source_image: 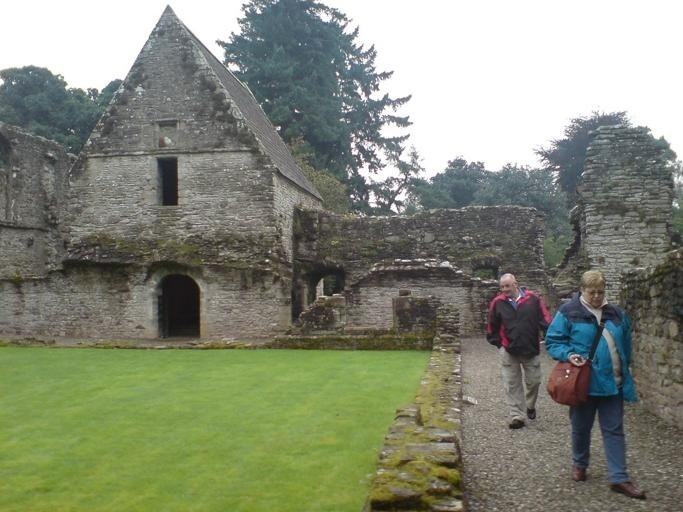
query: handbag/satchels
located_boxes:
[547,361,591,407]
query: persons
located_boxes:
[545,270,646,499]
[486,273,552,428]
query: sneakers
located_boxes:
[509,419,524,429]
[527,407,536,420]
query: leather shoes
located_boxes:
[571,464,586,482]
[608,481,645,499]
